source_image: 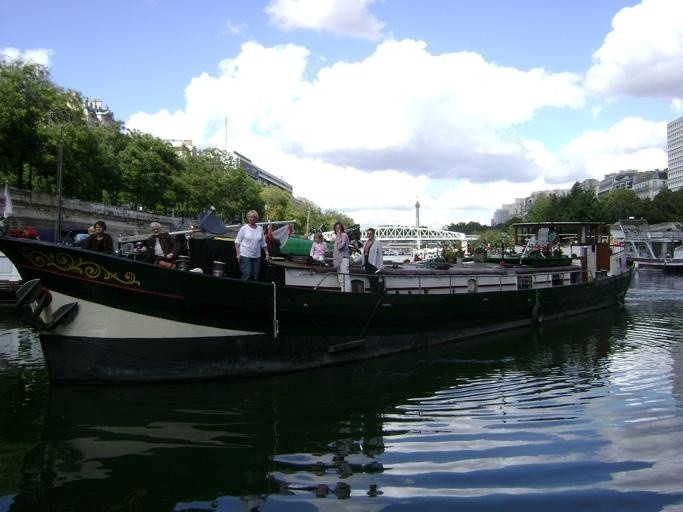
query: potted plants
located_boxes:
[484,230,513,268]
[446,252,455,263]
[456,250,464,265]
[473,247,487,262]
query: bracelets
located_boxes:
[264,251,268,255]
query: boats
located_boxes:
[0,223,637,384]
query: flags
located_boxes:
[2,185,13,220]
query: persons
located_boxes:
[233,209,271,280]
[74,224,95,245]
[360,228,384,293]
[330,222,352,293]
[304,405,388,501]
[306,230,328,268]
[136,221,175,267]
[70,221,114,256]
